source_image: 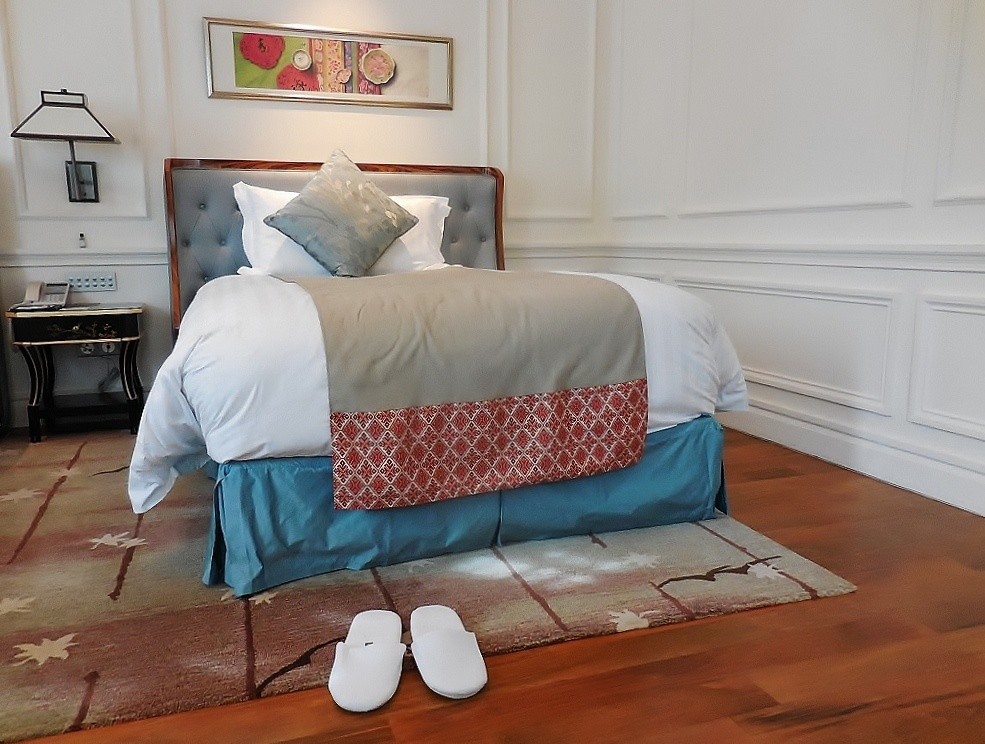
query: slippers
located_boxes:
[328,610,407,711]
[409,605,488,699]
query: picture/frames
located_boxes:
[202,16,455,112]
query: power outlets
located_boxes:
[75,343,100,359]
[99,342,120,356]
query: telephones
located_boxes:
[23,280,70,307]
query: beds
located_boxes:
[124,148,749,599]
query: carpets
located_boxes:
[0,417,862,744]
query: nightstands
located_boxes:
[4,302,147,444]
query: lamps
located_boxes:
[8,88,115,204]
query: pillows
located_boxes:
[231,180,452,285]
[262,148,420,278]
